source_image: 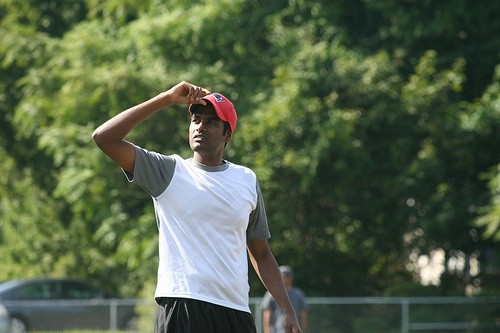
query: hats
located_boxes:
[279,265,294,274]
[189,93,238,134]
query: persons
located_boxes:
[91,81,302,333]
[259,265,308,333]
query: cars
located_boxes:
[0,277,141,333]
